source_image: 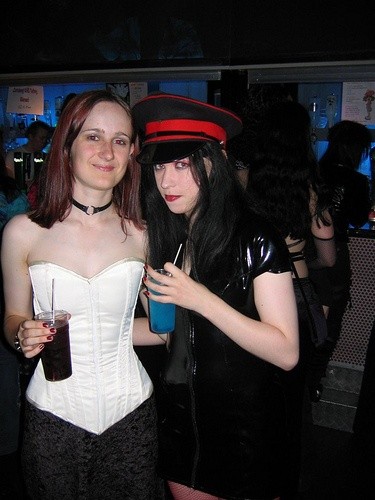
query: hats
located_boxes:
[130,92,245,167]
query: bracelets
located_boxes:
[14,323,24,355]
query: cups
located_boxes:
[146,269,176,334]
[33,310,72,381]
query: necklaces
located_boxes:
[68,191,114,215]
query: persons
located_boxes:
[0,154,31,231]
[225,82,294,190]
[235,96,335,500]
[0,90,171,500]
[308,119,372,402]
[4,122,49,181]
[132,96,300,500]
